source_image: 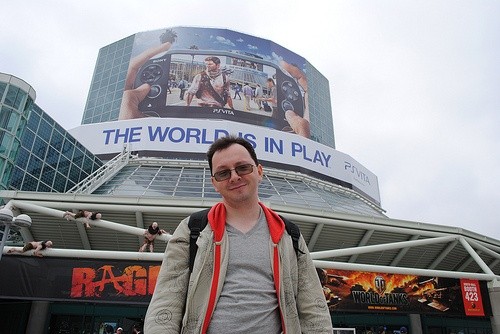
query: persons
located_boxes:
[367,326,407,334]
[167,77,188,100]
[143,137,333,334]
[118,41,310,139]
[186,57,235,109]
[100,324,143,334]
[243,82,254,111]
[234,83,242,100]
[63,210,102,228]
[7,240,52,256]
[139,222,166,252]
[255,78,277,116]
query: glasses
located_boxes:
[212,164,255,183]
